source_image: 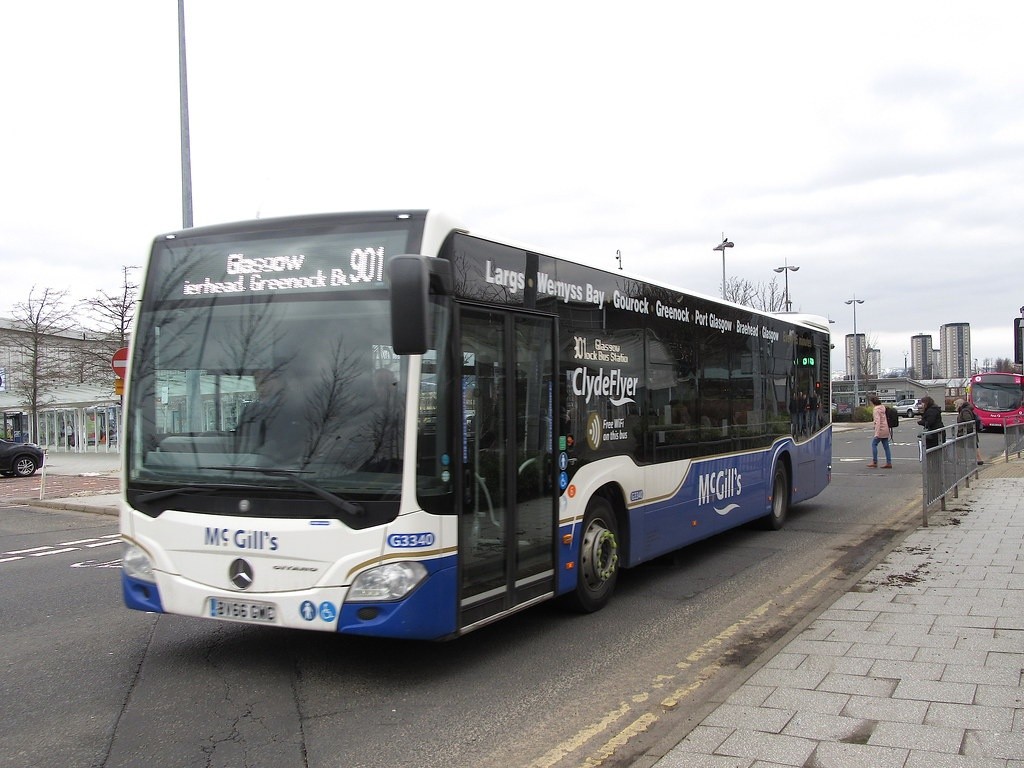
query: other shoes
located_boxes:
[977,460,984,465]
[866,459,877,467]
[881,462,892,469]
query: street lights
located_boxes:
[844,294,864,408]
[712,231,734,300]
[615,249,622,270]
[773,257,800,312]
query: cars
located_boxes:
[0,438,44,478]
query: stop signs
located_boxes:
[112,347,128,379]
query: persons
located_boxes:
[670,400,689,424]
[790,390,818,439]
[916,397,946,448]
[236,367,291,438]
[866,396,892,468]
[68,421,73,445]
[954,399,983,466]
[372,370,397,393]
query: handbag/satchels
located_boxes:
[885,406,898,428]
[917,423,931,440]
[974,413,981,431]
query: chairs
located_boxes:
[622,394,757,438]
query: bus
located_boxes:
[832,391,879,414]
[0,409,105,450]
[965,373,1024,434]
[116,206,833,636]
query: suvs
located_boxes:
[892,398,926,418]
